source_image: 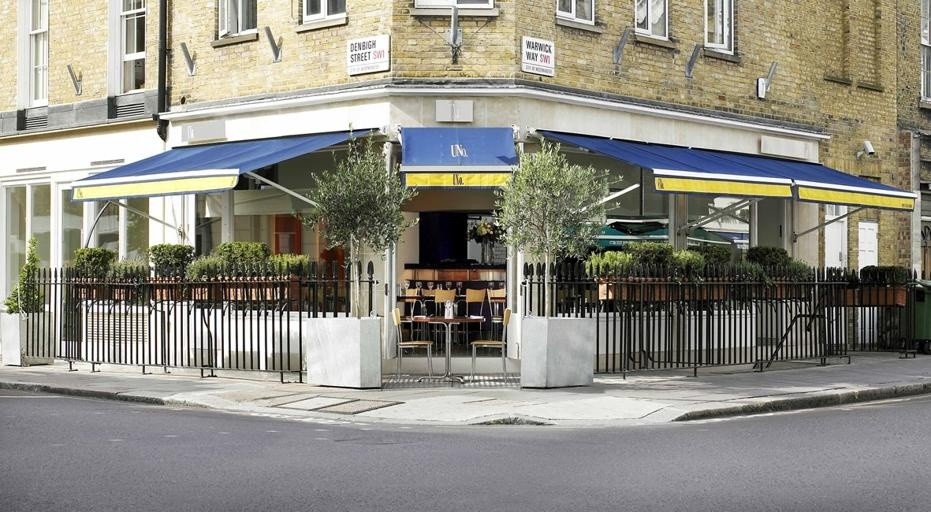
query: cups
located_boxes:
[498,282,505,289]
[416,282,422,288]
[437,283,443,290]
[403,281,410,289]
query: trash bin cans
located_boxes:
[900,278,931,355]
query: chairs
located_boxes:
[390,287,511,385]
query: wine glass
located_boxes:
[446,281,453,290]
[488,282,494,290]
[456,282,462,297]
[427,282,434,291]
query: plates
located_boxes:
[468,315,485,319]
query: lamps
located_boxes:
[263,25,281,64]
[66,63,82,96]
[756,61,777,100]
[612,27,630,66]
[449,5,461,64]
[684,44,702,79]
[178,42,196,77]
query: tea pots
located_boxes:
[444,300,454,320]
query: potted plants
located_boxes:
[490,138,622,390]
[305,125,422,392]
[1,239,53,367]
[73,241,316,303]
